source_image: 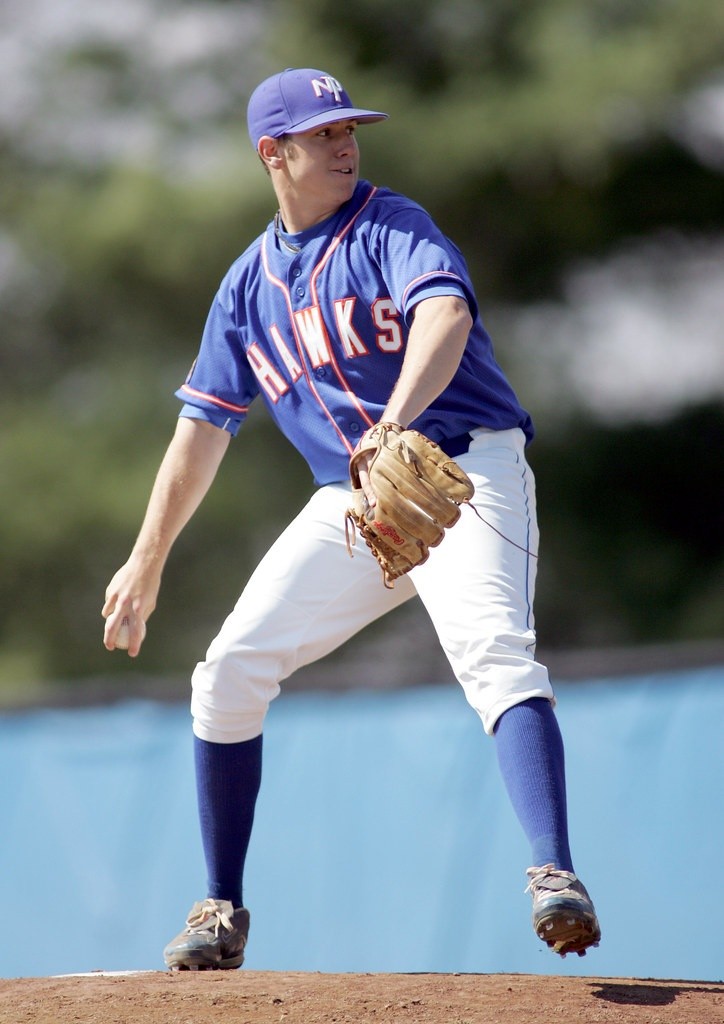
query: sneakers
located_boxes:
[525,864,601,959]
[163,900,250,972]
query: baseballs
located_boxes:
[106,613,146,650]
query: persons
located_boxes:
[101,68,600,972]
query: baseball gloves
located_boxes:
[341,424,476,591]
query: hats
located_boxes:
[247,68,388,150]
